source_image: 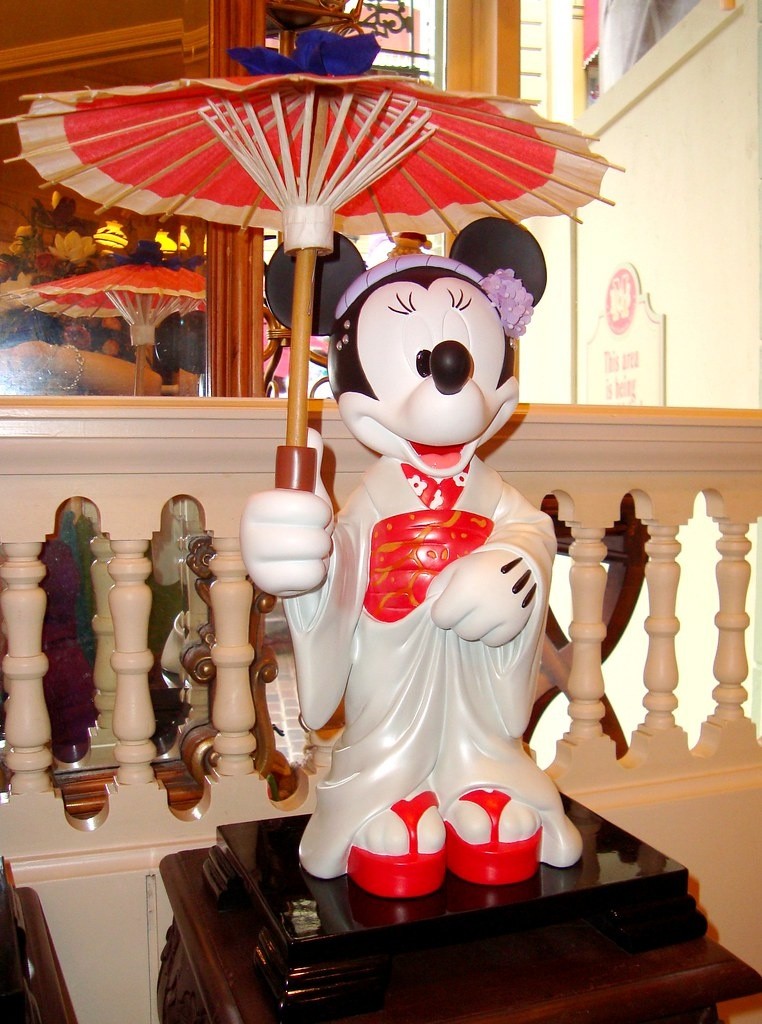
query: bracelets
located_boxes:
[29,344,85,391]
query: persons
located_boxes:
[1,306,161,395]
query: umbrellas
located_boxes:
[2,29,628,493]
[1,240,207,395]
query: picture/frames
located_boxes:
[572,0,742,144]
